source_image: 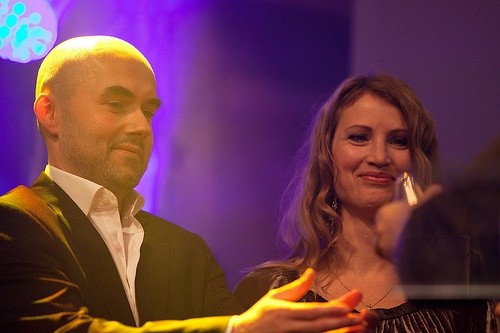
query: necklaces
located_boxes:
[328,256,408,308]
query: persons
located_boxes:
[375,137,499,315]
[233,74,499,333]
[0,33,377,332]
